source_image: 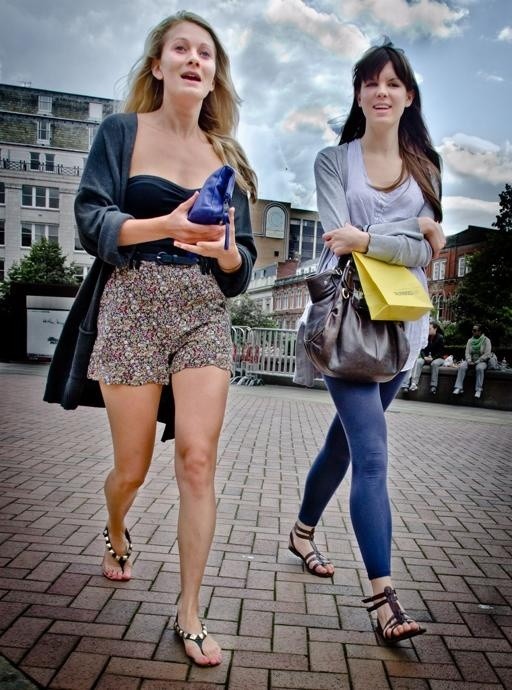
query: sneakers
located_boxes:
[401,381,439,396]
[452,387,481,399]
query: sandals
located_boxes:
[174,612,222,668]
[287,520,336,578]
[100,523,134,583]
[360,586,430,643]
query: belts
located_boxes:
[129,249,200,266]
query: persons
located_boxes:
[452,324,492,399]
[409,321,448,394]
[288,36,448,644]
[401,378,411,393]
[41,9,257,666]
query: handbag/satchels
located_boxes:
[186,164,237,228]
[350,249,434,322]
[487,352,501,370]
[302,244,410,385]
